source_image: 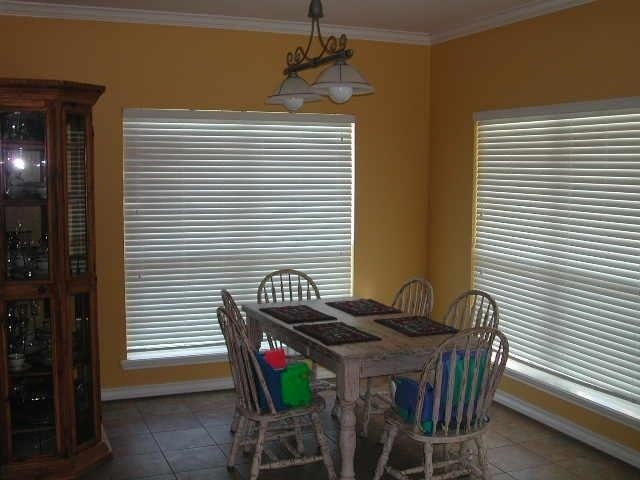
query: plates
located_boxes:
[5,363,32,375]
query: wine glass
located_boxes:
[5,297,53,363]
[3,218,51,283]
[2,109,51,199]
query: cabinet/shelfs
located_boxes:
[0,75,112,480]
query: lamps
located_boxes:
[264,0,375,112]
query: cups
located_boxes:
[263,349,288,372]
[8,353,26,369]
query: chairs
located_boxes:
[373,327,509,480]
[256,269,321,374]
[361,290,499,445]
[216,308,338,480]
[221,288,256,455]
[391,278,434,317]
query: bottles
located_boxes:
[11,384,52,427]
[12,430,56,459]
[72,311,90,391]
